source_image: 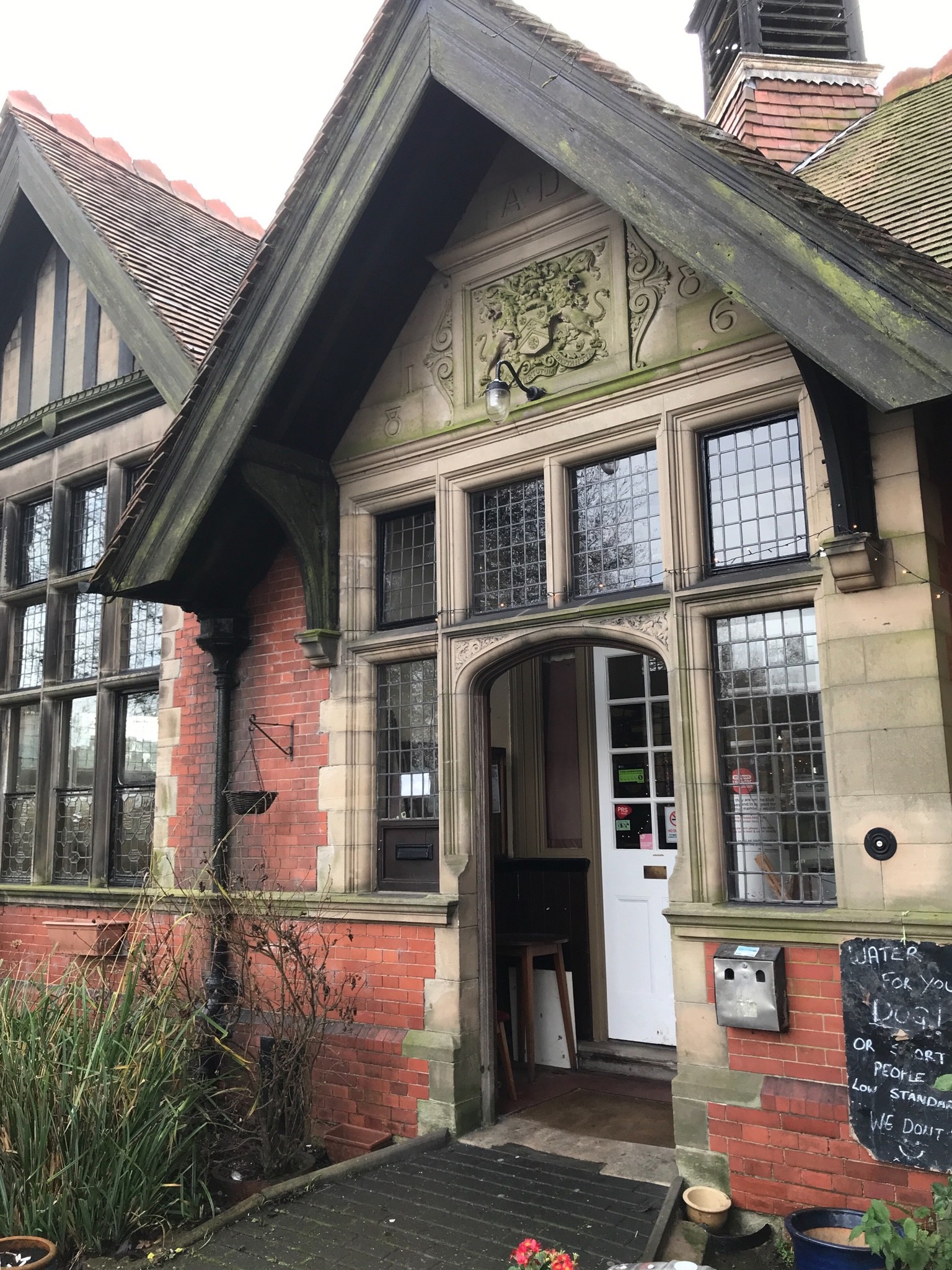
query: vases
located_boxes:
[786,1208,903,1269]
[683,1188,730,1231]
[326,1124,393,1163]
[42,918,128,956]
[0,1236,56,1270]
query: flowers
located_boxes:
[509,1235,578,1268]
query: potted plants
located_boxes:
[210,874,317,1198]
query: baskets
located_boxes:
[223,790,278,814]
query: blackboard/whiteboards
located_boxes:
[841,937,952,1175]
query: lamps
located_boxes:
[487,361,541,426]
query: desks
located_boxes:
[496,931,578,1084]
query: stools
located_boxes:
[496,1011,517,1100]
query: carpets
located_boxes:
[504,1086,675,1150]
